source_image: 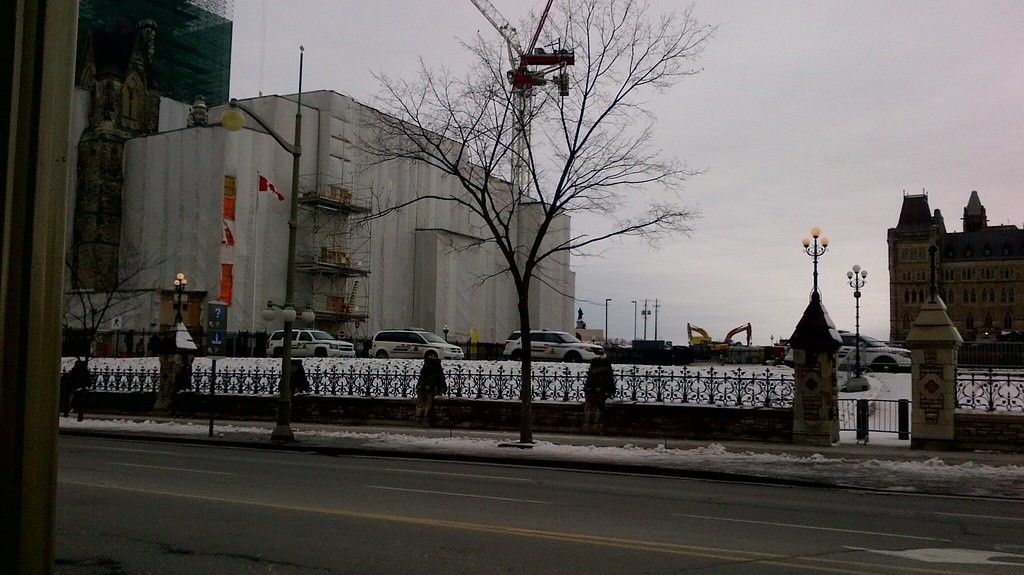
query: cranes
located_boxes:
[470,1,575,197]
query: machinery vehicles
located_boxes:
[686,322,752,360]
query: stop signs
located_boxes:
[338,330,346,339]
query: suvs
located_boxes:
[503,330,607,363]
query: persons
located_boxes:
[720,348,725,366]
[576,332,581,342]
[124,329,176,358]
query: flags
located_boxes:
[256,173,285,201]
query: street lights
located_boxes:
[847,264,870,392]
[631,301,637,341]
[172,273,188,326]
[801,227,829,302]
[605,298,611,350]
[221,45,315,443]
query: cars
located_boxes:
[957,341,1024,365]
[783,329,912,373]
[368,328,465,361]
[265,327,356,357]
